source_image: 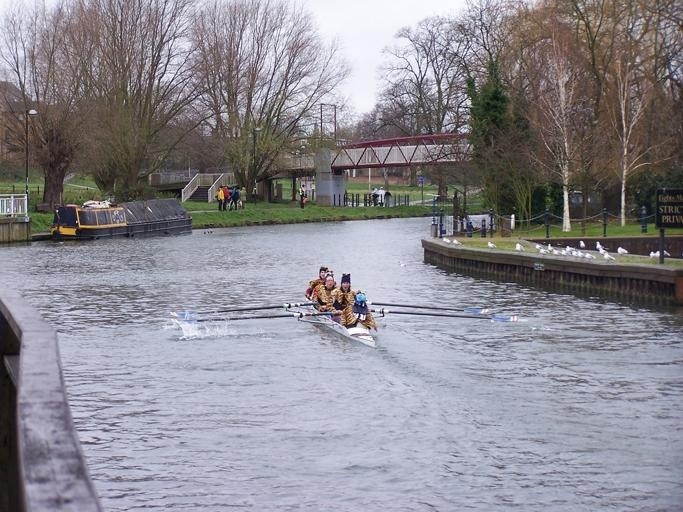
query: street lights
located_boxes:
[250,127,261,205]
[23,108,38,213]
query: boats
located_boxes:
[51,197,193,240]
[303,296,379,351]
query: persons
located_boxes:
[306,267,375,329]
[299,184,306,208]
[251,185,258,205]
[217,184,248,211]
[370,186,392,207]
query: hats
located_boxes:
[341,274,350,282]
[356,293,366,302]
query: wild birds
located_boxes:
[595,241,609,250]
[452,239,463,247]
[535,240,596,260]
[515,243,526,253]
[617,246,629,256]
[603,251,615,263]
[442,237,452,246]
[649,250,671,260]
[487,242,498,250]
[599,247,608,255]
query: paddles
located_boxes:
[366,301,490,314]
[178,302,318,318]
[369,308,518,323]
[184,311,340,324]
